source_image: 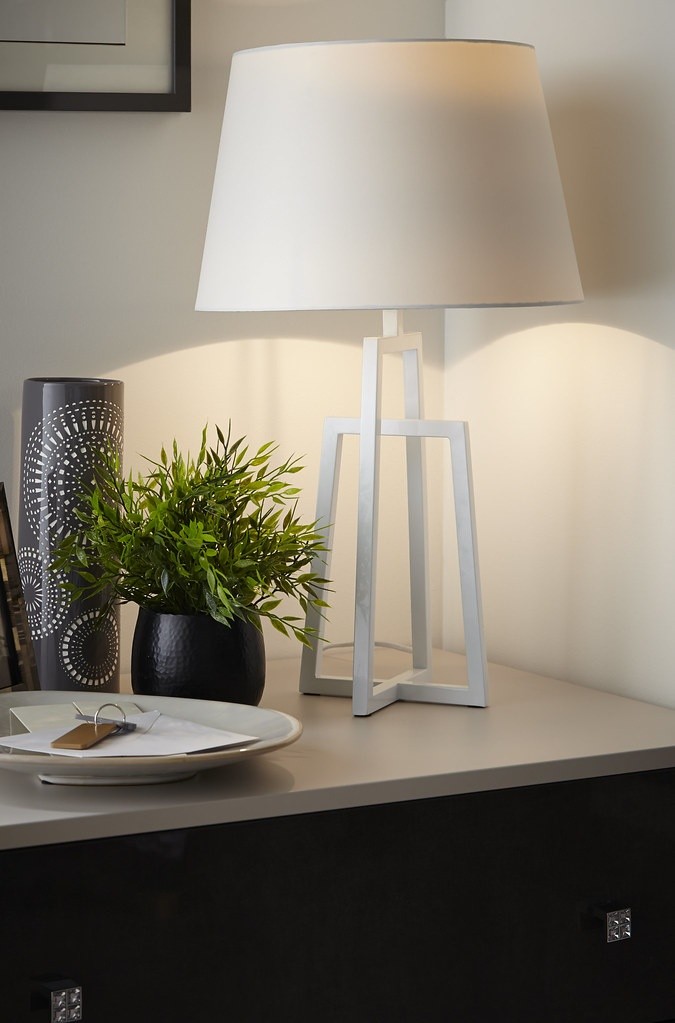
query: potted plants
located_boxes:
[47,420,337,708]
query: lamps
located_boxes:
[192,39,582,718]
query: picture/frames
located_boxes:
[0,0,193,113]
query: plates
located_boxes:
[1,691,304,786]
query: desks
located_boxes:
[0,641,675,1023]
